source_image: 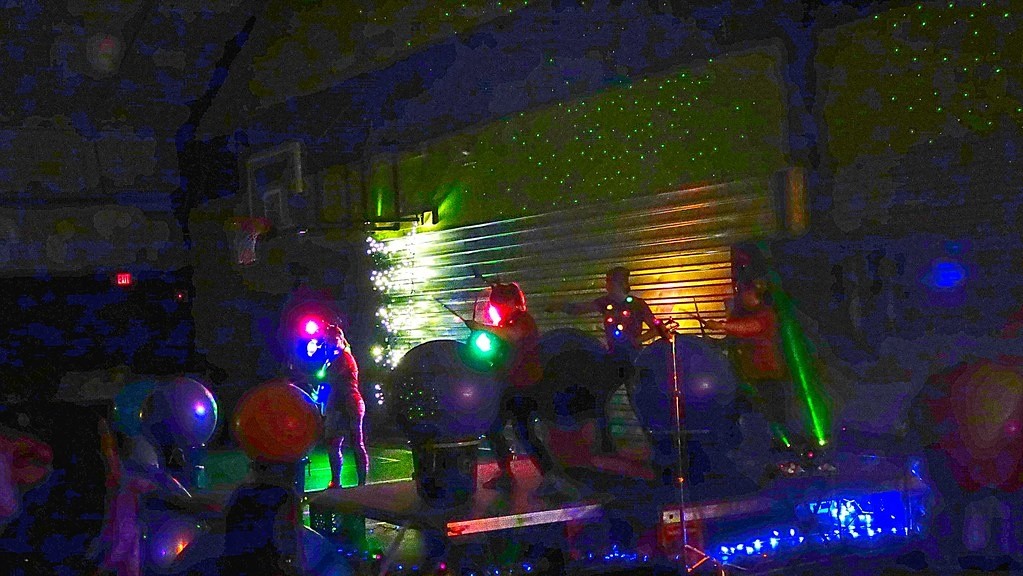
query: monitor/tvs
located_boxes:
[248,139,308,242]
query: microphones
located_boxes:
[636,321,672,347]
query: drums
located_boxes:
[408,441,480,509]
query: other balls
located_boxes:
[147,372,218,447]
[386,340,506,444]
[232,378,324,462]
[632,331,733,434]
[532,330,613,424]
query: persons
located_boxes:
[545,268,672,452]
[465,283,567,496]
[18,403,197,576]
[217,458,306,576]
[288,326,368,490]
[706,276,836,473]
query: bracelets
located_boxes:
[719,320,726,330]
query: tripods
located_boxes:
[662,333,756,576]
